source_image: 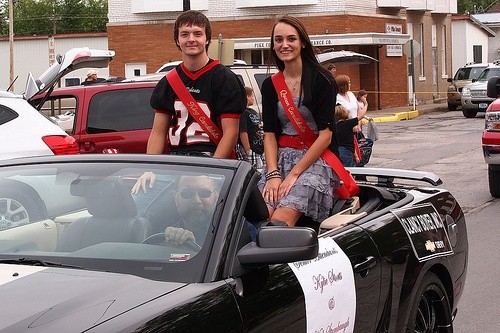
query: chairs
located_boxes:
[57,178,269,252]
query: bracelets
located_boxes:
[265,170,282,180]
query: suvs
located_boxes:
[447,62,496,111]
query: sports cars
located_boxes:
[0,153,469,333]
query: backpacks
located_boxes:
[356,131,373,167]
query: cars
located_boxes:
[120,48,378,134]
[27,81,175,154]
[0,47,115,161]
[461,60,500,118]
[481,77,500,197]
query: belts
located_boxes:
[278,135,309,150]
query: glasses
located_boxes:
[181,189,215,199]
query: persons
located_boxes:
[328,65,336,75]
[356,91,371,133]
[333,77,365,166]
[130,10,246,218]
[333,99,370,168]
[161,175,259,247]
[255,15,338,227]
[81,70,100,85]
[237,87,266,169]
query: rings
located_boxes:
[265,190,270,192]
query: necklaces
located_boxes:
[286,74,301,90]
